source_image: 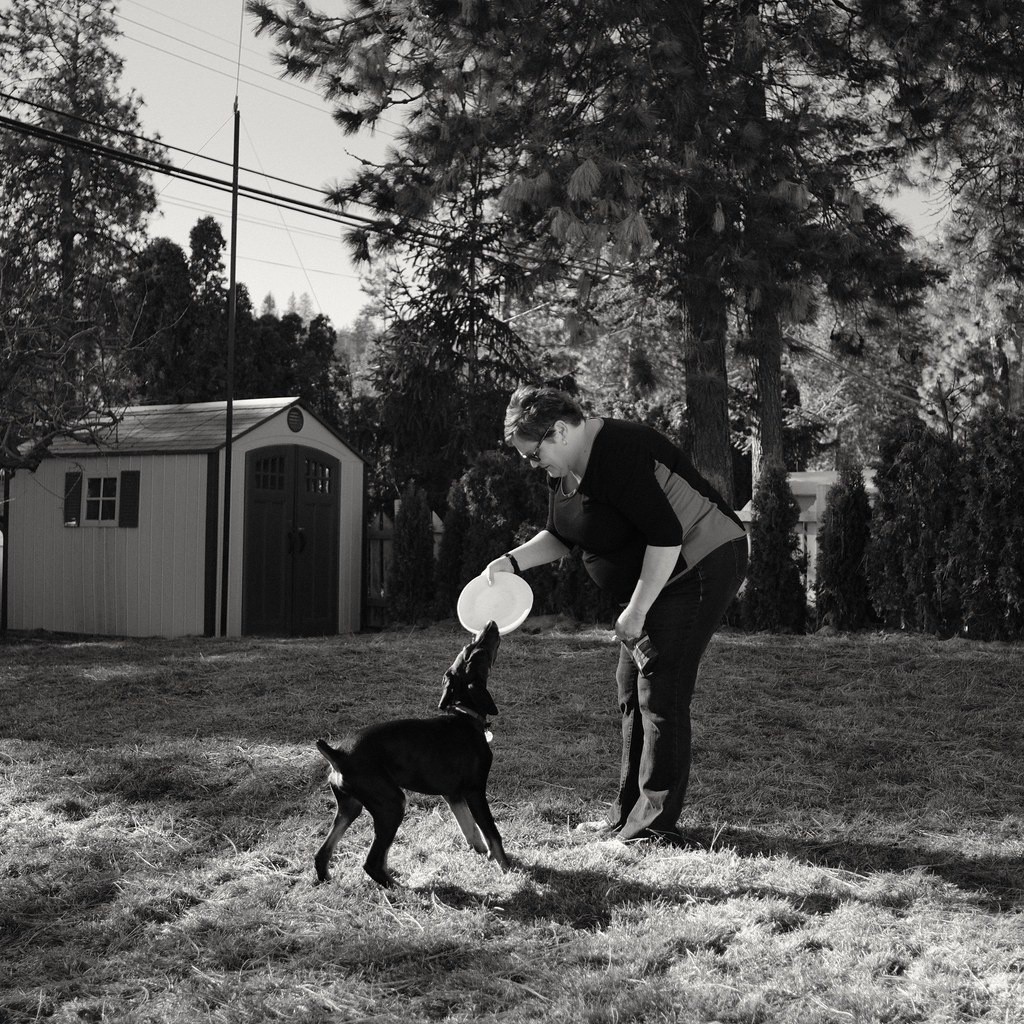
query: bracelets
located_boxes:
[503,553,522,574]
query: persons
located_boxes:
[479,384,749,853]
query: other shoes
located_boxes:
[576,820,611,836]
[596,840,630,855]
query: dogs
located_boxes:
[313,619,515,893]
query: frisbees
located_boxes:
[456,572,533,636]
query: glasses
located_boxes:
[522,423,555,464]
